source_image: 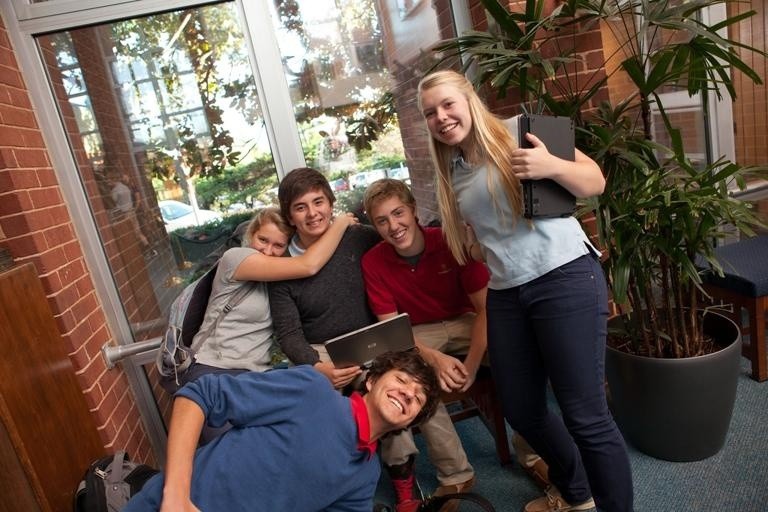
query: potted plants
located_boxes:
[420,0,768,463]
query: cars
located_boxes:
[324,167,413,193]
[159,199,222,235]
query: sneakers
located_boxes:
[433,475,474,512]
[390,456,420,512]
[523,459,597,512]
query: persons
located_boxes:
[364,178,555,511]
[179,209,354,443]
[116,350,441,512]
[416,70,633,510]
[99,169,160,259]
[270,169,424,512]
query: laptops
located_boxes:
[517,114,576,220]
[324,312,416,369]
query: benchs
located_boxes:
[687,234,768,382]
[412,355,514,473]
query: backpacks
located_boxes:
[156,259,220,392]
[74,451,160,512]
